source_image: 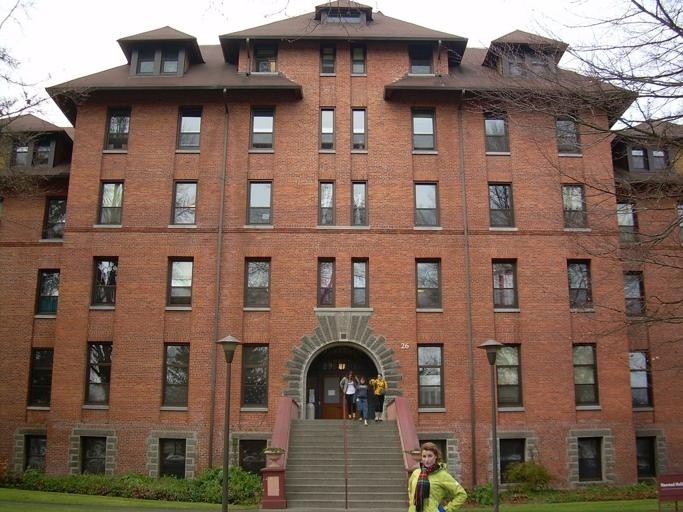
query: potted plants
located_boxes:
[400,448,422,468]
[263,447,287,467]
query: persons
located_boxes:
[405,442,468,512]
[368,373,387,421]
[354,375,372,425]
[339,370,360,418]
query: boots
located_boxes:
[374,411,383,421]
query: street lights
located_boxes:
[475,339,507,512]
[215,333,245,512]
